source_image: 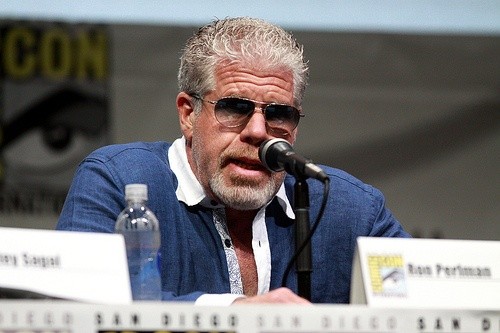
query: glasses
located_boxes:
[187,93,306,135]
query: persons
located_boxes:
[56,17,413,306]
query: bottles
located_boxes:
[114,184,162,301]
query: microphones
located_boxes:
[258,138,330,184]
